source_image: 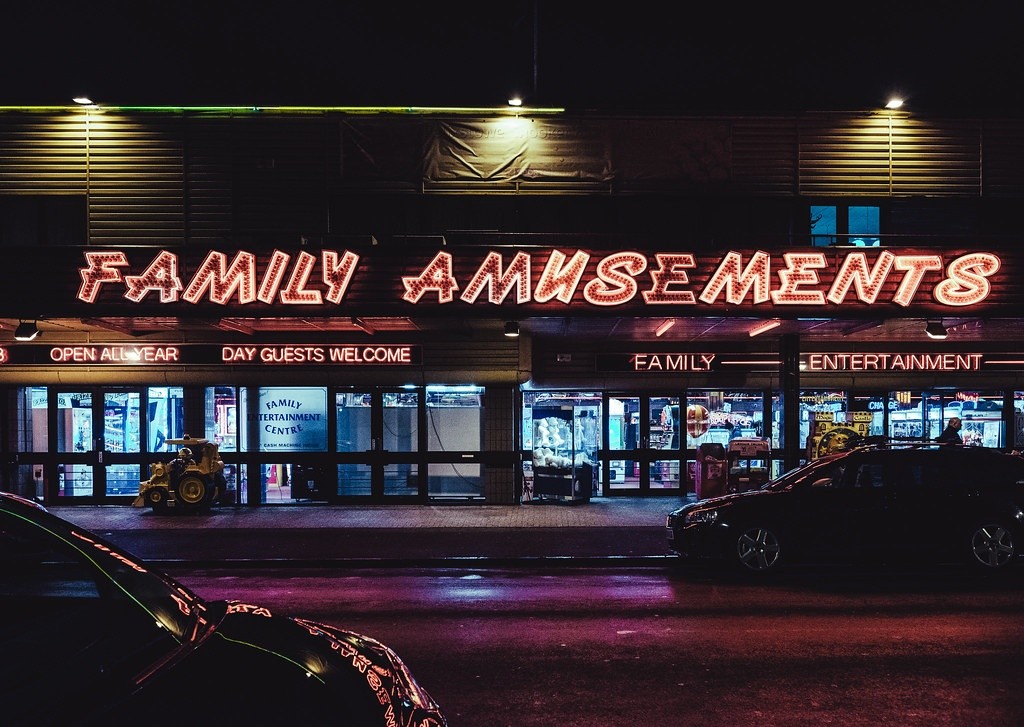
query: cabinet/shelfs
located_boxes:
[727,436,772,495]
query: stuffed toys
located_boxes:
[533,417,598,467]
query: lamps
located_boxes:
[924,317,948,340]
[749,320,781,337]
[655,319,676,337]
[14,320,38,341]
[505,321,520,337]
[72,95,95,106]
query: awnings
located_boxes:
[0,311,428,334]
[523,316,1024,342]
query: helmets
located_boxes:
[179,448,193,455]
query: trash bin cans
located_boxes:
[695,443,728,500]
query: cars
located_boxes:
[0,493,446,727]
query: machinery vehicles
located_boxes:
[132,434,227,515]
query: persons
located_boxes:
[155,430,165,452]
[170,448,196,472]
[725,423,742,448]
[937,417,964,444]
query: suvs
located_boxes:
[664,442,1024,585]
